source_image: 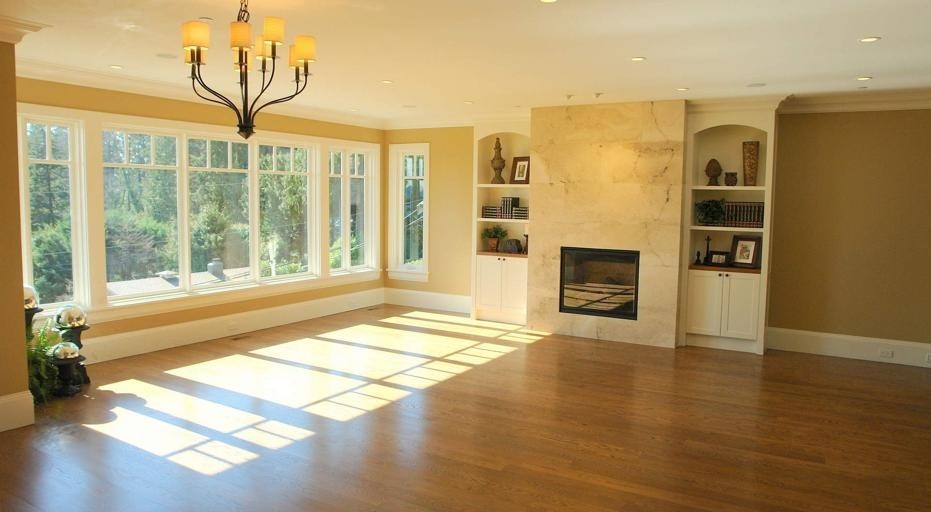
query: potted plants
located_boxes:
[482,227,507,252]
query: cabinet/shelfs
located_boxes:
[472,182,528,257]
[686,268,761,341]
[475,254,526,316]
[689,184,765,275]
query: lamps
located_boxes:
[178,0,316,141]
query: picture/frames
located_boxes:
[509,156,529,185]
[706,234,761,270]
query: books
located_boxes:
[724,200,764,228]
[482,195,529,220]
